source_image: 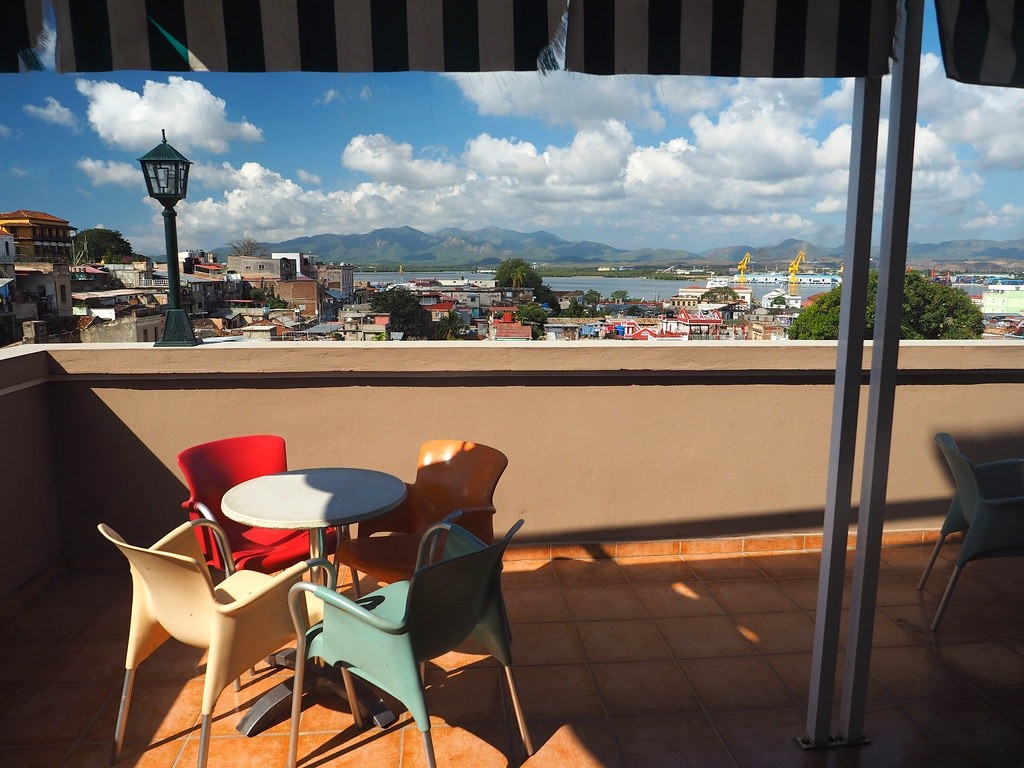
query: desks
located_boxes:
[219,468,408,737]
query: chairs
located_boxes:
[331,440,508,690]
[916,434,1024,628]
[97,521,362,768]
[174,434,360,677]
[287,519,533,768]
